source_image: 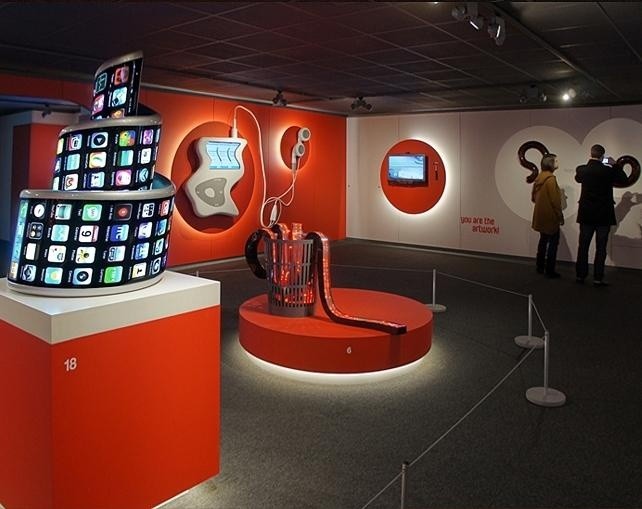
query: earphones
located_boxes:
[291,142,305,171]
[297,127,311,144]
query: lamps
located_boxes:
[432,1,508,44]
[514,80,599,104]
[343,95,375,114]
[270,89,288,108]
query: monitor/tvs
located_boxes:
[387,154,427,185]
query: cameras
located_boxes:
[602,158,609,164]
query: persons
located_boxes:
[530,153,564,279]
[575,144,629,286]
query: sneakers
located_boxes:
[535,268,544,274]
[544,272,562,279]
[592,279,613,288]
[576,276,587,284]
